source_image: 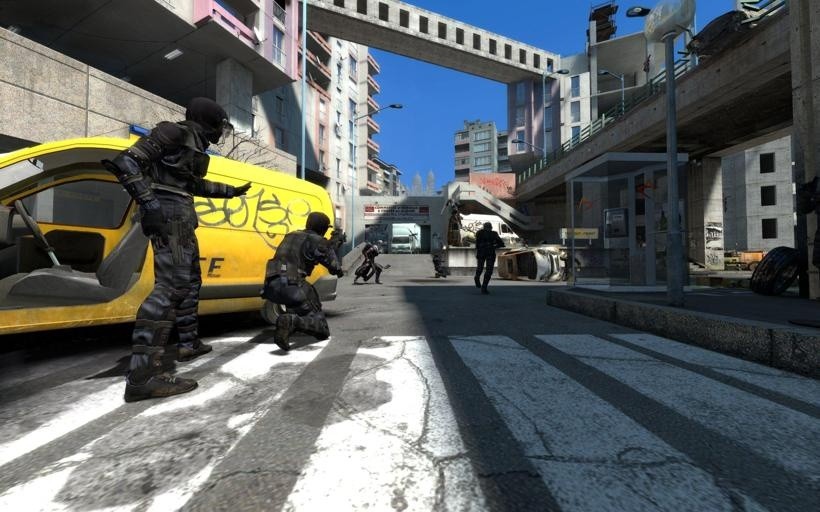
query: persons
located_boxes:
[473,221,505,294]
[264,212,346,351]
[100,97,251,403]
[353,239,383,283]
